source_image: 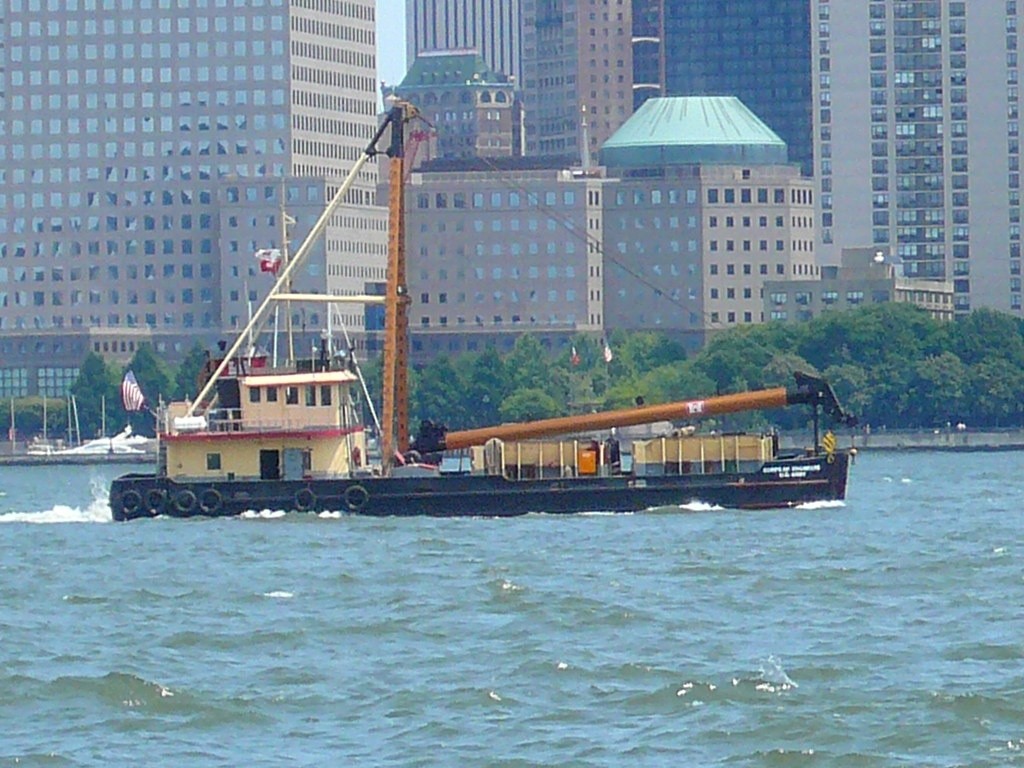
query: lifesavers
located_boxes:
[292,488,317,512]
[197,488,223,516]
[343,484,370,511]
[173,489,198,516]
[118,490,143,518]
[143,488,169,516]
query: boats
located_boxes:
[111,93,860,517]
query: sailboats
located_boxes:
[9,397,158,462]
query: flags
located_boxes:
[604,344,612,362]
[122,370,145,411]
[823,432,836,452]
[571,347,579,365]
[256,249,279,261]
[260,260,281,273]
